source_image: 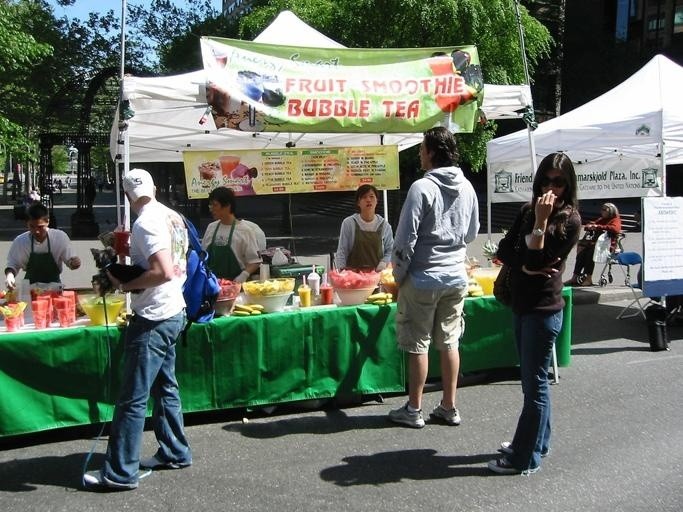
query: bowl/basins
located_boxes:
[215,281,241,316]
[332,286,378,304]
[247,291,295,312]
[379,282,400,301]
[77,293,124,324]
[475,268,498,294]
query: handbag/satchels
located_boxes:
[493,208,533,306]
[175,208,221,323]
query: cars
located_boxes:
[0,172,77,189]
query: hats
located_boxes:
[123,168,156,201]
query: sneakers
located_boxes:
[500,441,514,454]
[434,401,461,424]
[488,458,526,474]
[389,403,425,428]
[139,456,169,469]
[82,469,109,490]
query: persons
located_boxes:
[237,216,267,252]
[329,185,394,274]
[562,203,621,289]
[81,169,191,491]
[389,126,482,428]
[51,175,72,195]
[199,188,263,285]
[3,203,80,287]
[485,153,582,477]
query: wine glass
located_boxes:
[212,78,240,132]
[428,57,461,134]
[236,65,265,133]
[258,65,289,122]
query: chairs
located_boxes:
[575,226,629,287]
[614,251,661,322]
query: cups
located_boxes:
[219,156,239,190]
[307,277,319,296]
[297,289,311,308]
[259,264,270,280]
[13,278,33,323]
[319,287,334,304]
[4,304,75,330]
[198,166,213,187]
[111,230,131,254]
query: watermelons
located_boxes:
[6,291,75,332]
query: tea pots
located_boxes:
[32,282,64,300]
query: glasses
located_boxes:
[538,176,566,188]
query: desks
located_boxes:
[0,284,578,441]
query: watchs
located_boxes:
[530,224,545,238]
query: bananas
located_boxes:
[364,291,393,305]
[232,304,264,316]
[468,288,484,297]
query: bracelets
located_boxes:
[116,282,125,294]
